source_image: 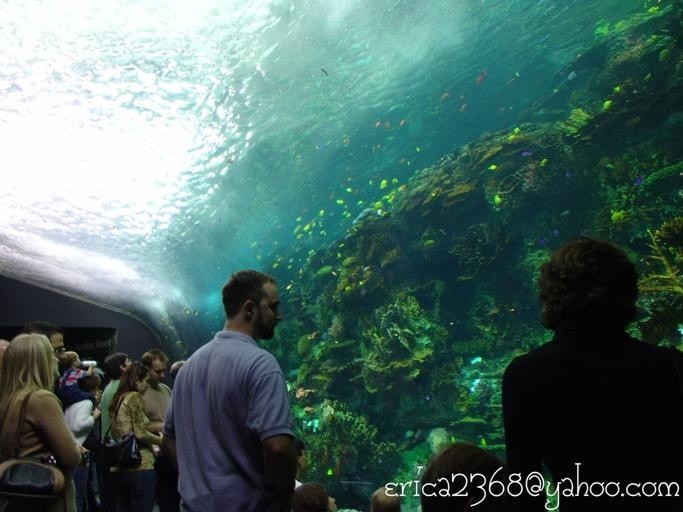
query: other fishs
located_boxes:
[250,67,630,296]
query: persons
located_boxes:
[158,266,301,511]
[293,482,338,512]
[500,231,682,511]
[419,442,507,511]
[0,317,187,510]
[294,436,308,491]
[370,486,402,512]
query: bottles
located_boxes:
[78,360,97,367]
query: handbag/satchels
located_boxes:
[0,388,67,502]
[97,396,142,469]
[82,414,101,453]
